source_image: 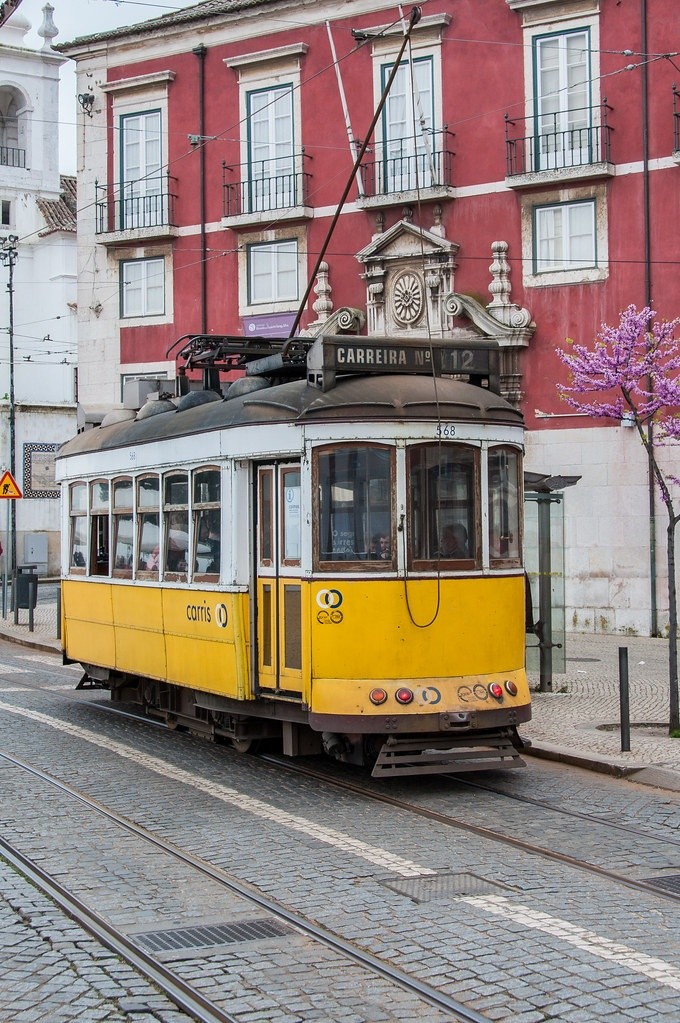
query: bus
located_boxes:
[56,330,534,780]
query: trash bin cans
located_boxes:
[17,565,38,609]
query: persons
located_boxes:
[145,510,221,573]
[439,523,469,560]
[371,534,391,560]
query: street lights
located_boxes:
[0,235,19,611]
